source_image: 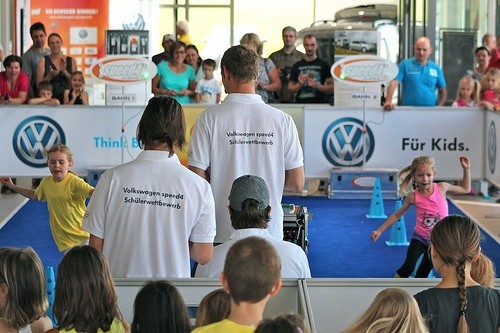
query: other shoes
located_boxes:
[479,185,500,197]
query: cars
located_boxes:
[348,40,374,53]
[335,38,349,49]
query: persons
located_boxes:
[174,21,191,45]
[63,72,91,105]
[240,33,282,103]
[452,34,500,113]
[404,215,500,333]
[371,156,471,280]
[195,174,310,279]
[131,237,308,333]
[82,99,216,278]
[286,34,333,103]
[265,26,306,103]
[381,35,448,112]
[44,244,131,333]
[150,34,205,103]
[194,58,222,105]
[0,247,53,333]
[342,287,429,333]
[0,22,76,106]
[0,143,97,256]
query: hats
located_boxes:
[228,175,270,212]
[163,34,176,43]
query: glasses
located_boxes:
[173,51,185,54]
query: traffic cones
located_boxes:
[384,200,411,247]
[44,266,58,329]
[365,176,389,220]
